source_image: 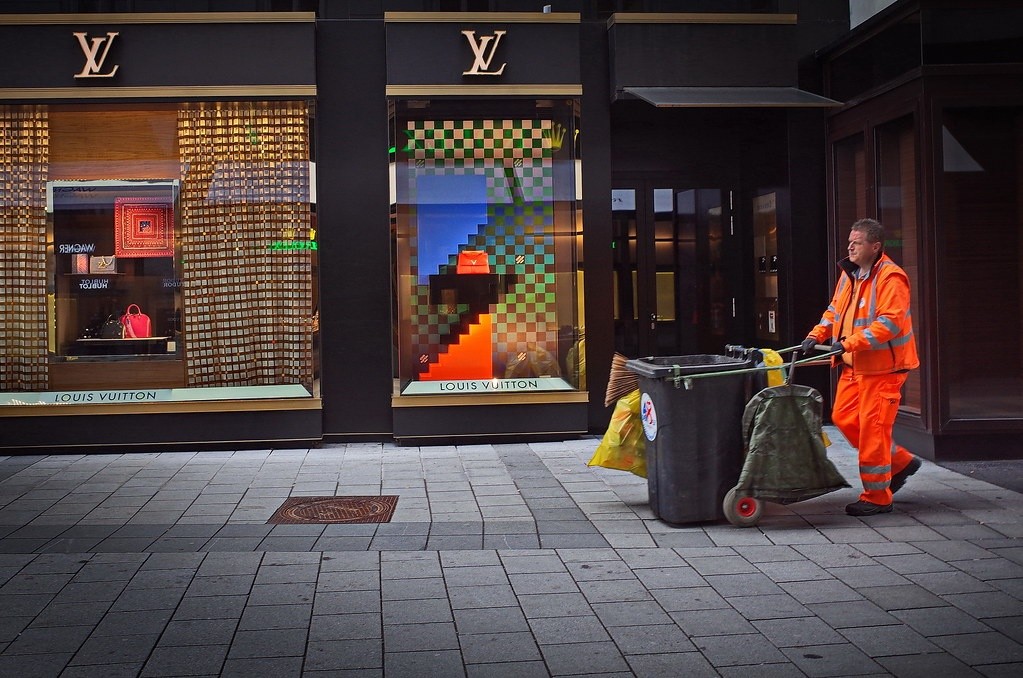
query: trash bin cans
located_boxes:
[624,344,765,528]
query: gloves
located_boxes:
[830,343,845,356]
[802,339,816,355]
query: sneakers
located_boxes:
[888,457,922,495]
[846,500,893,516]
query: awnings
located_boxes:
[624,87,847,108]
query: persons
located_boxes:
[802,216,921,516]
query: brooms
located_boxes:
[605,351,831,408]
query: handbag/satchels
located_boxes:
[101,313,123,339]
[72,255,89,274]
[120,304,152,338]
[457,251,489,274]
[90,255,118,273]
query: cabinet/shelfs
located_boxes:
[63,272,168,345]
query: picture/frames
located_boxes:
[115,196,175,258]
